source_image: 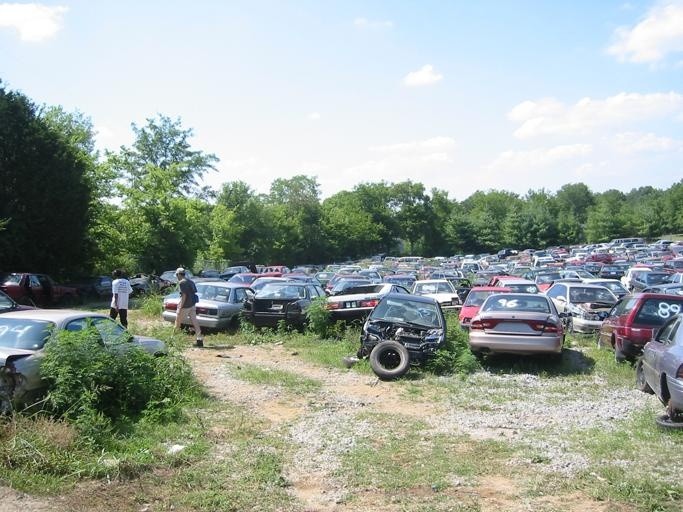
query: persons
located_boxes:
[109,269,133,328]
[171,267,204,347]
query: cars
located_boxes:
[0,308,168,417]
[0,272,166,312]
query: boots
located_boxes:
[193,340,204,347]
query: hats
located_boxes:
[175,268,186,275]
